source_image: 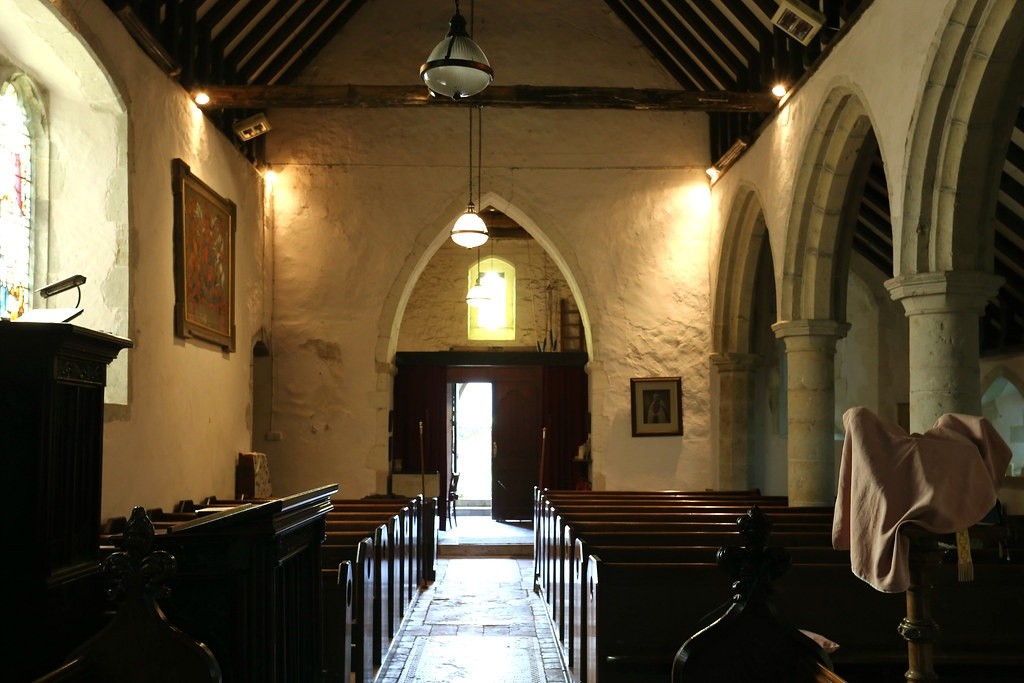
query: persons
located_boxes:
[647,394,666,424]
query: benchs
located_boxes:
[530,485,1024,683]
[97,494,437,683]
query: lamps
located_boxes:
[419,0,496,101]
[769,0,839,48]
[231,111,272,141]
[448,1,490,251]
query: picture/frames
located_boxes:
[630,377,685,438]
[171,158,238,354]
